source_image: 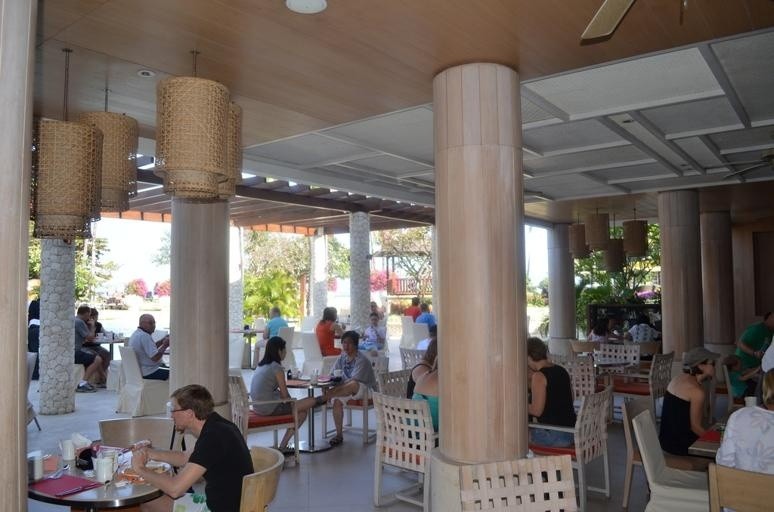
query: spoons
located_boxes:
[47,463,70,479]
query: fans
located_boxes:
[580,1,691,41]
[702,149,774,181]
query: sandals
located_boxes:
[330,436,343,447]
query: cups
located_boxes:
[744,397,757,406]
[58,432,124,485]
[96,331,125,341]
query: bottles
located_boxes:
[285,365,346,386]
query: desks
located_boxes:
[278,372,339,454]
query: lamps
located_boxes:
[623,207,649,257]
[219,101,243,203]
[567,212,591,258]
[155,51,228,203]
[605,212,625,272]
[585,208,609,249]
[33,47,103,241]
[77,59,138,213]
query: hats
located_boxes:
[684,347,721,368]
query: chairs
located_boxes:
[461,454,585,511]
[115,346,169,418]
[706,462,773,511]
[320,356,390,443]
[620,396,692,508]
[239,446,285,511]
[225,373,301,463]
[527,386,613,497]
[374,316,440,436]
[632,412,713,511]
[527,325,772,460]
[370,388,439,511]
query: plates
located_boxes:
[121,460,171,477]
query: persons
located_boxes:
[526,337,576,448]
[659,347,721,455]
[716,309,773,510]
[252,308,288,369]
[132,384,254,512]
[243,325,255,336]
[129,315,169,379]
[588,315,661,366]
[404,297,439,433]
[75,307,102,391]
[251,337,316,452]
[85,308,117,385]
[315,301,385,445]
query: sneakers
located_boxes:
[77,382,97,393]
[278,446,294,456]
